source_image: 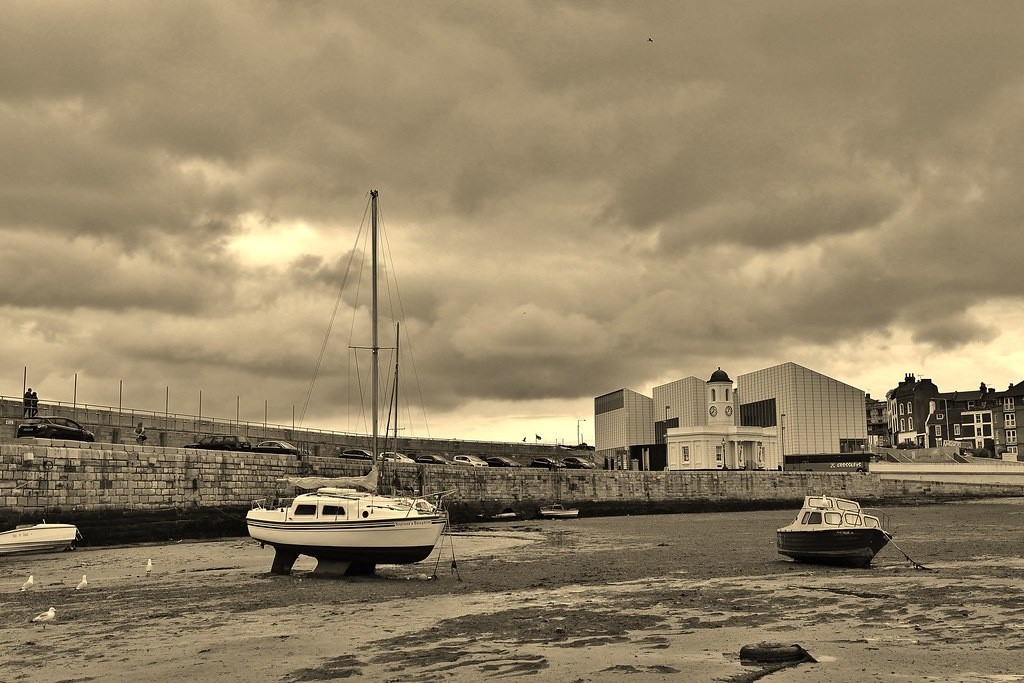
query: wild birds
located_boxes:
[647,38,653,43]
[146,559,153,571]
[29,607,57,628]
[76,575,87,590]
[19,575,33,592]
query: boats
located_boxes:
[538,504,580,518]
[773,496,892,563]
[0,521,79,557]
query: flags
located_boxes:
[536,434,541,440]
[666,405,670,409]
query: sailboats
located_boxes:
[245,188,449,566]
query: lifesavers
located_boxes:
[741,641,802,659]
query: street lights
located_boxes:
[577,419,586,445]
[665,405,670,429]
[721,437,728,470]
[931,397,949,440]
[781,413,785,470]
[860,445,866,472]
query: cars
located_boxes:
[485,456,523,467]
[338,449,373,459]
[16,416,95,443]
[453,454,489,468]
[257,441,313,457]
[416,454,459,466]
[379,452,416,463]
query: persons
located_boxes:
[21,388,39,419]
[548,456,559,472]
[134,422,145,446]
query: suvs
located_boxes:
[561,457,594,469]
[530,457,567,468]
[182,436,251,453]
